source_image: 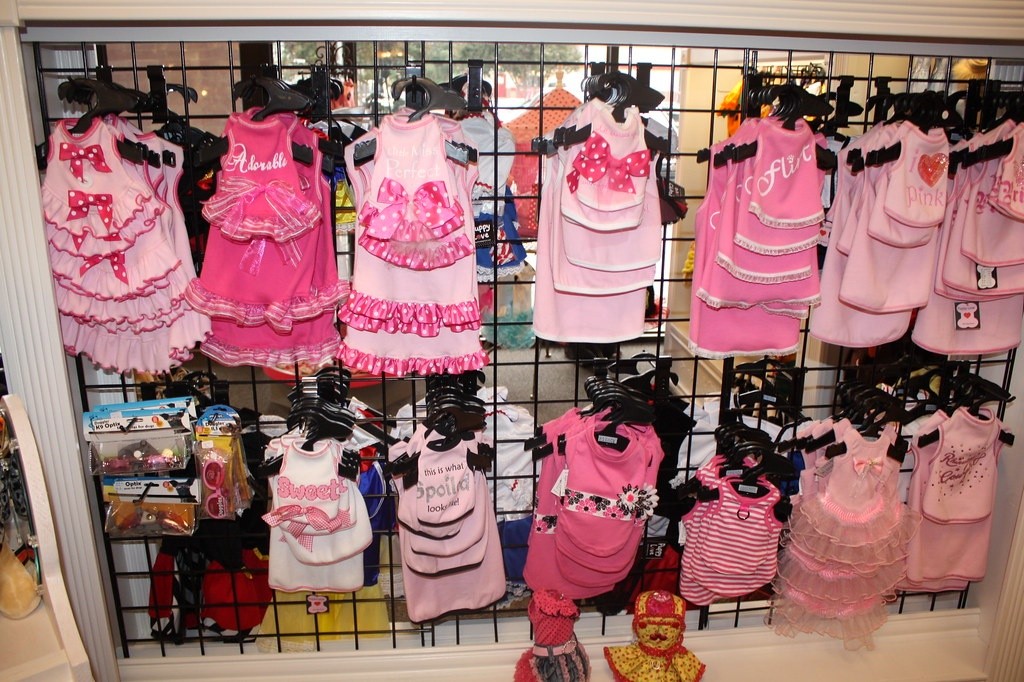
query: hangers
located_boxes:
[32,61,1024,176]
[90,357,1016,521]
[87,351,1014,520]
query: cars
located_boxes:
[651,101,680,179]
